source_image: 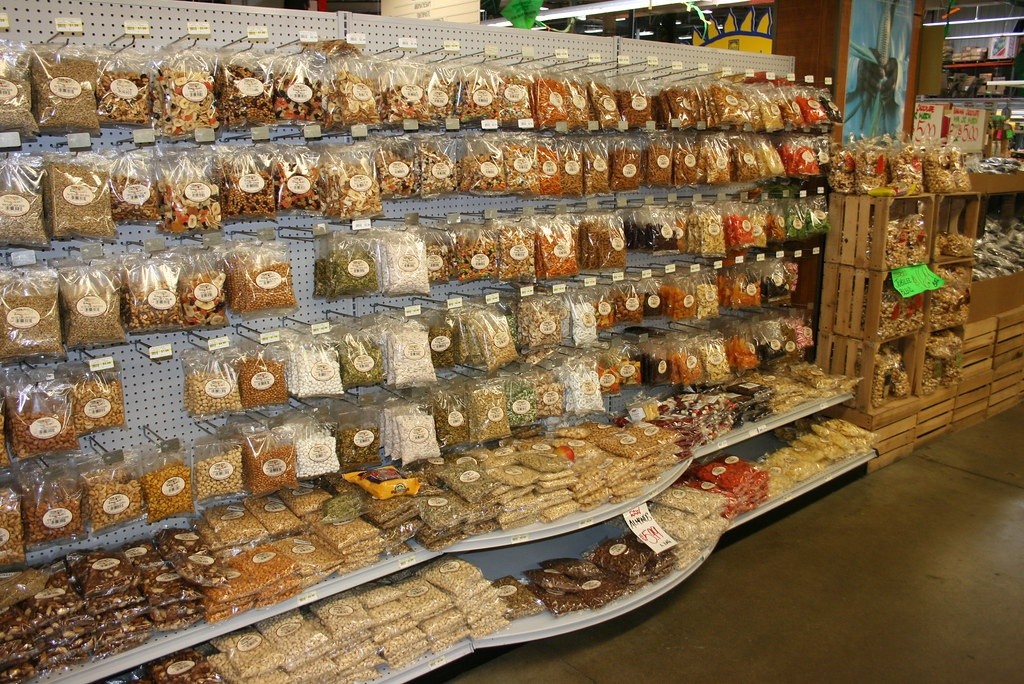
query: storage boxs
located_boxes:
[978,73,1007,90]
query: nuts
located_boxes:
[189,490,387,625]
[0,376,196,564]
[206,558,513,684]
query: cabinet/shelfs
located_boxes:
[0,0,875,684]
[942,61,1015,98]
[815,174,1023,432]
